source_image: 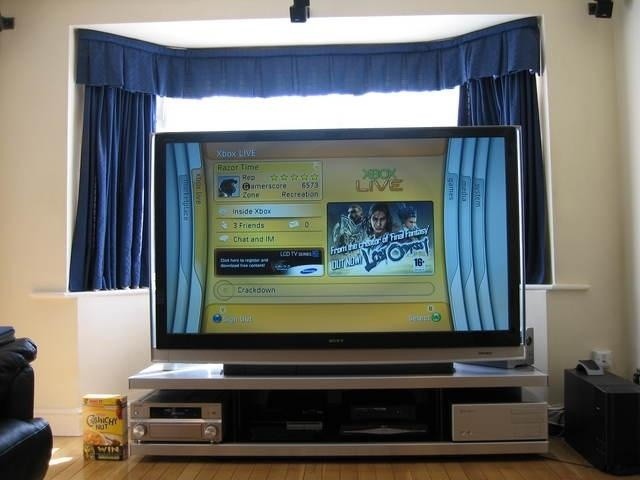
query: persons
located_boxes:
[345,203,364,225]
[398,203,422,232]
[363,203,395,240]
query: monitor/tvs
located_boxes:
[151,125,526,375]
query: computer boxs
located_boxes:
[564,366,639,472]
